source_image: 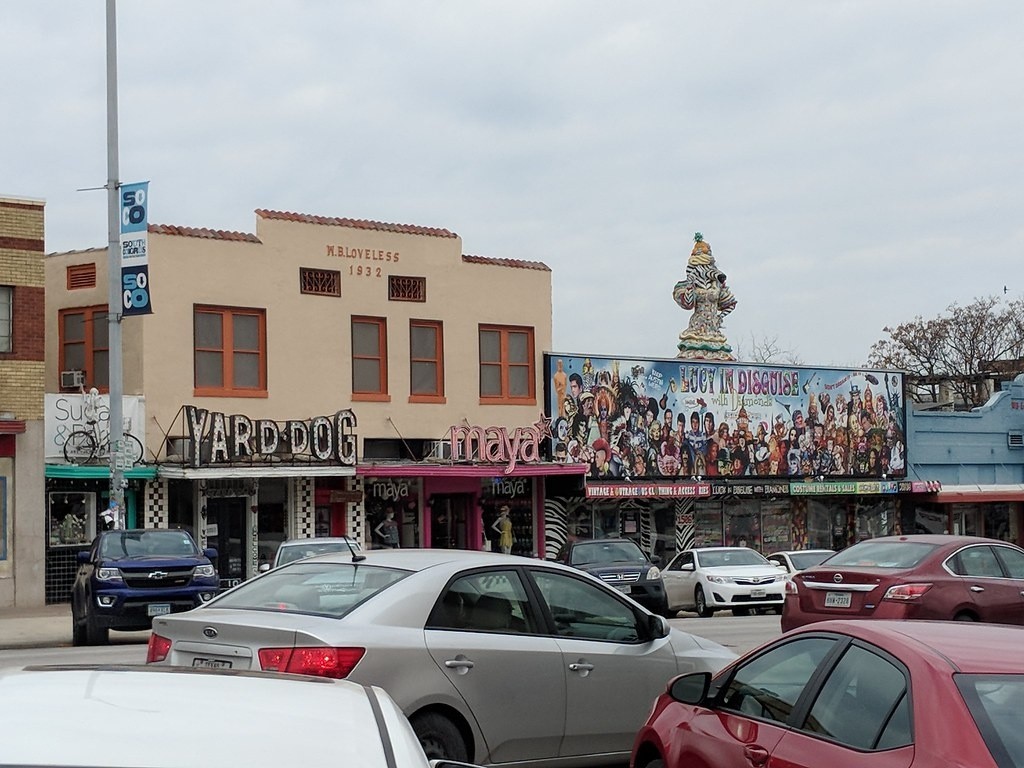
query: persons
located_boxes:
[491,506,513,554]
[374,508,399,549]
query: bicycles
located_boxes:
[64,418,143,466]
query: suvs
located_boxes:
[555,535,668,618]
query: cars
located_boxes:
[260,535,367,607]
[627,620,1024,768]
[765,549,836,580]
[144,548,739,768]
[781,533,1024,635]
[661,546,787,618]
[70,529,221,647]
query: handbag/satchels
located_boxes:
[512,530,518,544]
[483,531,491,551]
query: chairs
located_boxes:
[275,585,319,610]
[442,591,514,630]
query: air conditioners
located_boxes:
[62,371,84,388]
[432,441,462,461]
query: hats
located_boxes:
[384,508,398,514]
[500,506,512,514]
